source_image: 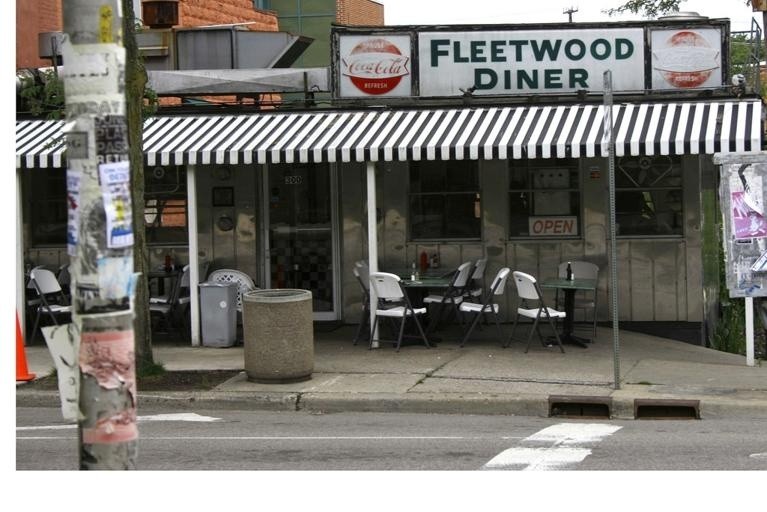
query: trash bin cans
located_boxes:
[198,281,236,348]
[242,289,314,383]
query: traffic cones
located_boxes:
[16,307,36,381]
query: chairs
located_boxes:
[352,258,599,355]
[22,264,261,346]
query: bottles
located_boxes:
[411,272,419,282]
[567,261,572,281]
[430,253,438,269]
[164,251,172,270]
[420,248,428,272]
